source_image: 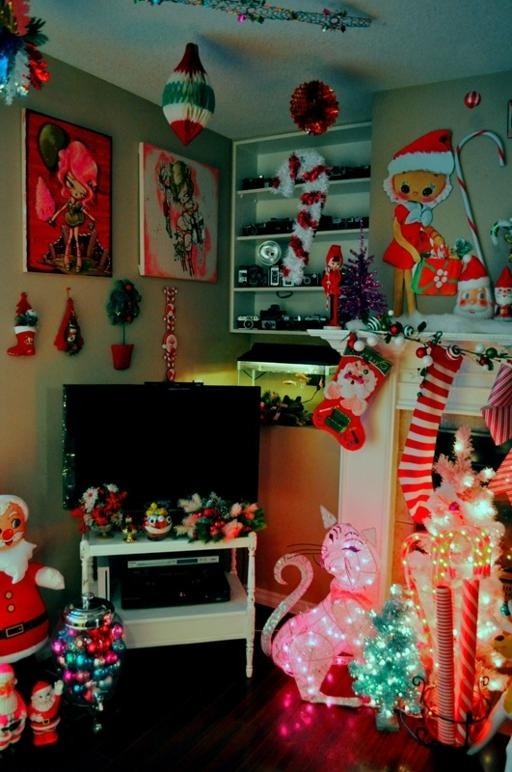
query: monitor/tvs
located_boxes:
[62,384,261,529]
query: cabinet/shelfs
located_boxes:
[228,120,372,336]
[79,530,258,677]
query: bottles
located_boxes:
[50,593,127,712]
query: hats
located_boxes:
[387,127,455,176]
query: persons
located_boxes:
[453,256,512,320]
[0,495,66,751]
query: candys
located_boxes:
[50,608,126,712]
[435,578,480,748]
